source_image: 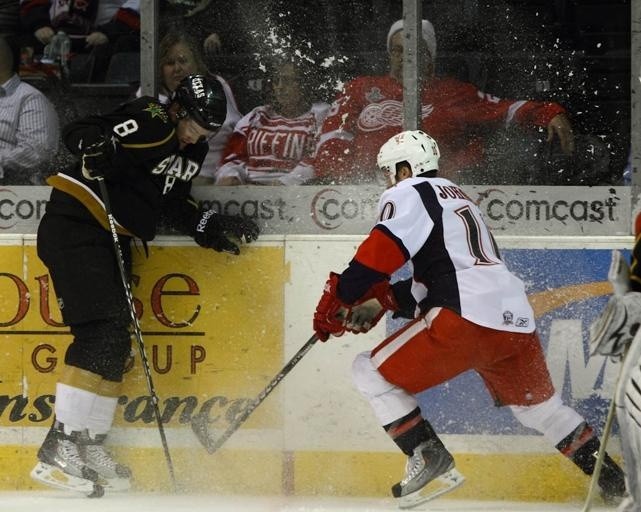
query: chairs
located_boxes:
[9,29,134,120]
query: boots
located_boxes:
[36,427,133,485]
[592,472,632,507]
[391,434,456,497]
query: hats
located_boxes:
[386,19,439,64]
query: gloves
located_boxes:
[312,272,402,343]
[172,202,259,256]
[81,134,121,185]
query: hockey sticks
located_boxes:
[191,329,319,454]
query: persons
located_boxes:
[312,131,634,507]
[1,0,246,184]
[35,71,263,486]
[315,18,574,182]
[589,191,640,512]
[215,58,330,186]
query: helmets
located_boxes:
[163,71,228,145]
[376,128,441,187]
